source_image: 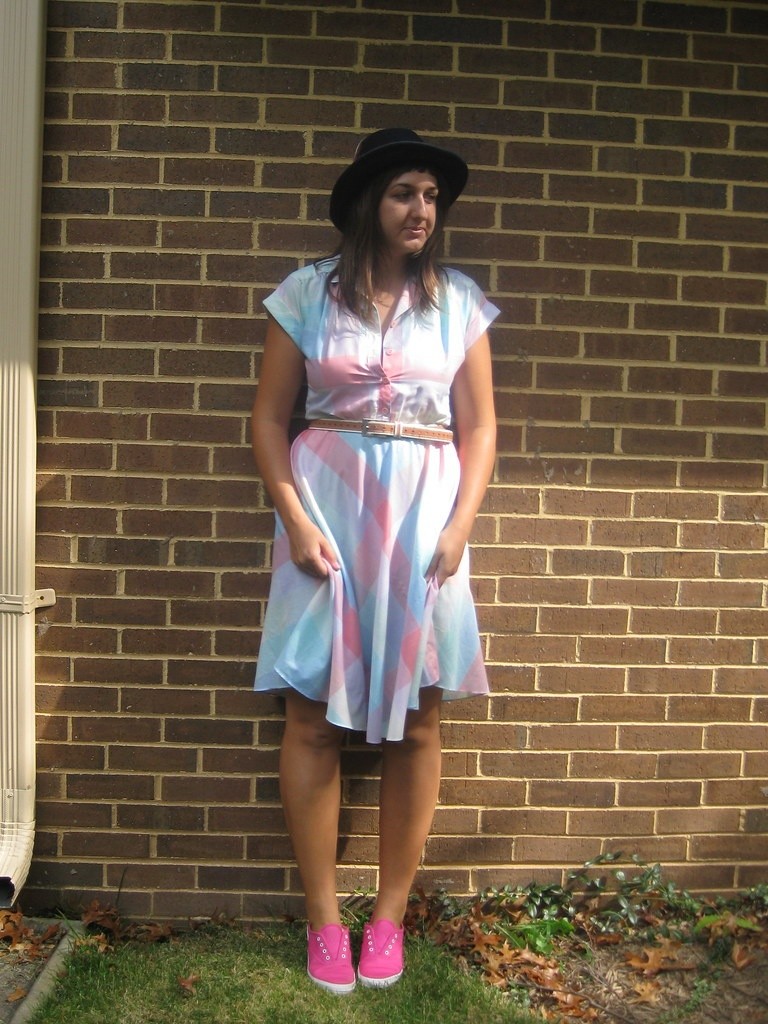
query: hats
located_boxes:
[329,127,469,235]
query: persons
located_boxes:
[252,129,503,993]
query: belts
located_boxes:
[307,418,455,444]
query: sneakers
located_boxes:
[306,921,355,994]
[357,912,405,987]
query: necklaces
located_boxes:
[374,297,396,310]
